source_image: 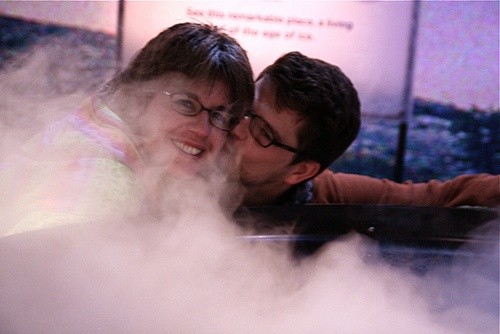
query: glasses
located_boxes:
[245,110,307,155]
[157,89,240,131]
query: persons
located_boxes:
[0,17,255,239]
[215,51,500,206]
